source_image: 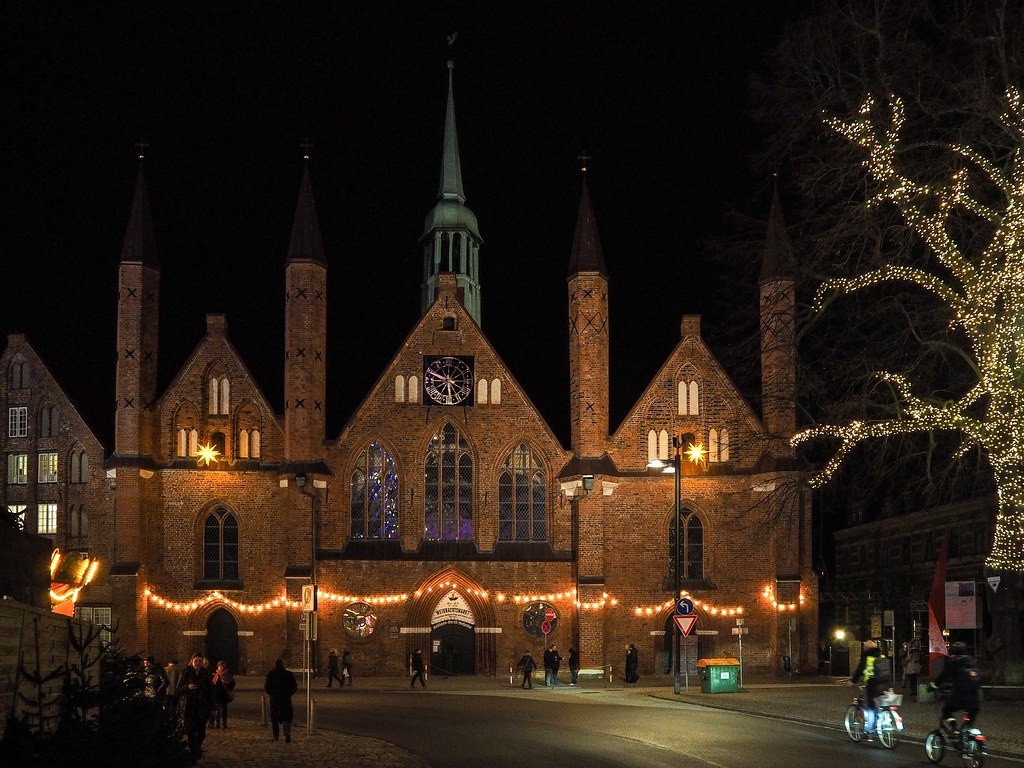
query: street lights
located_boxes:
[646,436,682,696]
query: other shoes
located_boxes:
[285,739,291,743]
[866,733,874,742]
[275,737,279,741]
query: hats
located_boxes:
[864,640,877,648]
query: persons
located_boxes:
[264,659,298,743]
[567,647,580,685]
[326,648,344,687]
[846,640,890,735]
[341,649,352,684]
[543,645,564,685]
[410,649,425,687]
[517,650,538,689]
[927,643,984,728]
[203,658,236,727]
[899,641,921,696]
[142,656,182,704]
[624,644,640,683]
[175,650,217,760]
[930,647,943,668]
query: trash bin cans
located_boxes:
[697,658,741,694]
[915,677,942,701]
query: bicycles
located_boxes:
[924,688,988,768]
[843,683,904,750]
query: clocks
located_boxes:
[423,353,476,407]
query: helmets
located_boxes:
[948,642,967,655]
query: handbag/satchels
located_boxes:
[228,692,235,701]
[522,666,527,671]
